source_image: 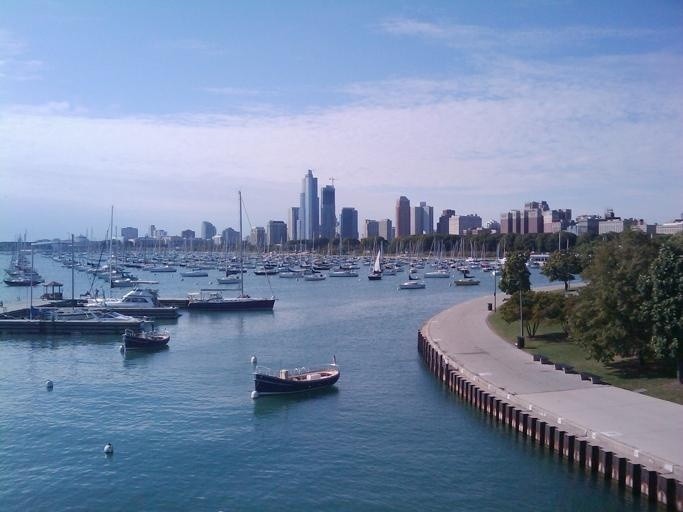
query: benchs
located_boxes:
[530,353,549,364]
[581,372,603,384]
[554,362,574,373]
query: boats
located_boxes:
[123,328,170,353]
[252,355,339,395]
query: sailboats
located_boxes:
[185,190,280,312]
[398,239,426,289]
[0,219,570,286]
[453,239,481,285]
[77,205,183,319]
[0,234,156,335]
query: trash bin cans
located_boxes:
[518,336,525,348]
[489,303,492,311]
